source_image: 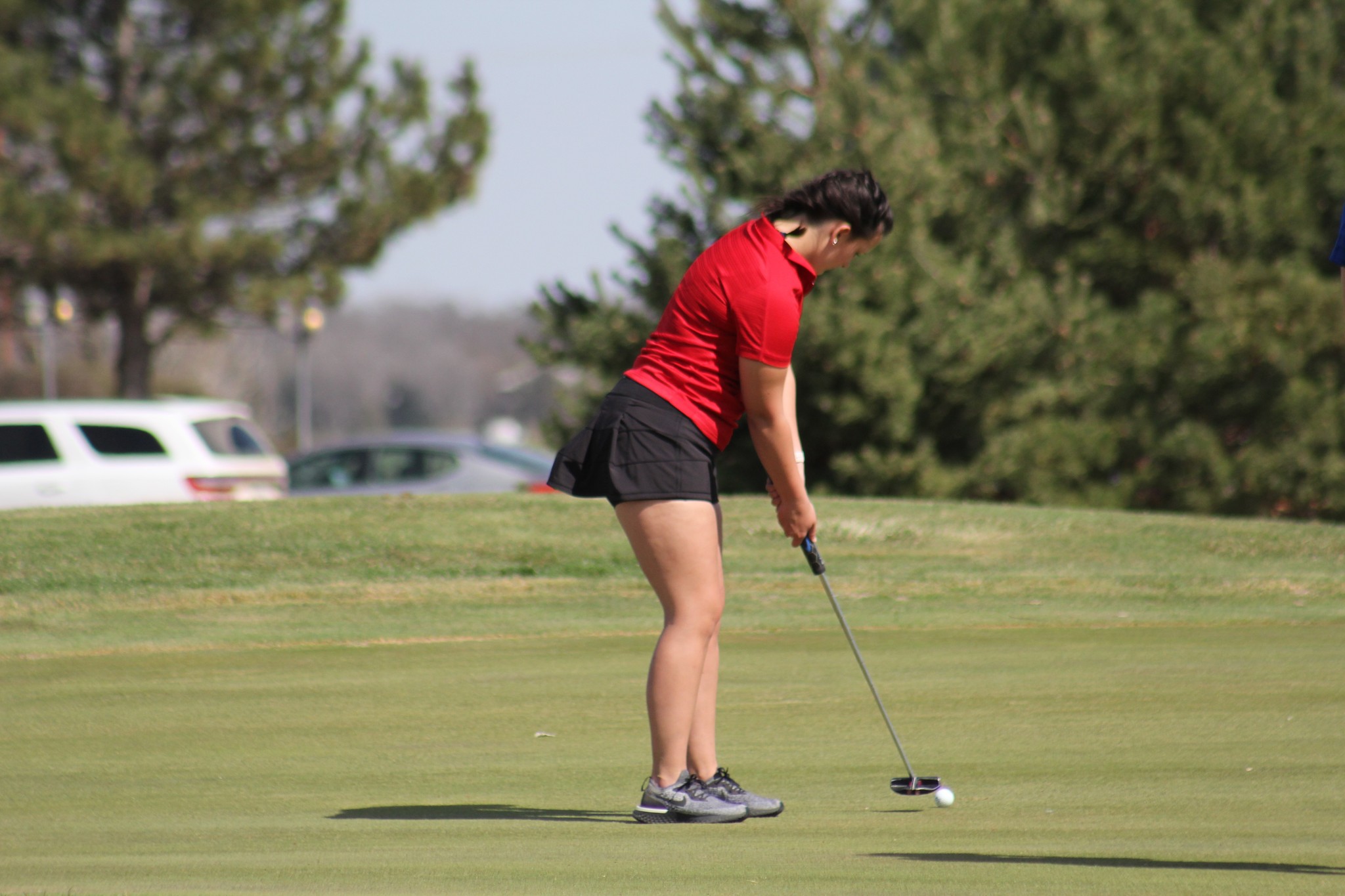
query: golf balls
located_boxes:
[934,788,955,808]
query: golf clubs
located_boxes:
[798,532,943,797]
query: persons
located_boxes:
[546,169,895,825]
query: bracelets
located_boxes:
[796,451,805,463]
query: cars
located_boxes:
[0,397,289,506]
[284,433,569,494]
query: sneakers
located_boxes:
[699,768,784,818]
[632,770,748,823]
